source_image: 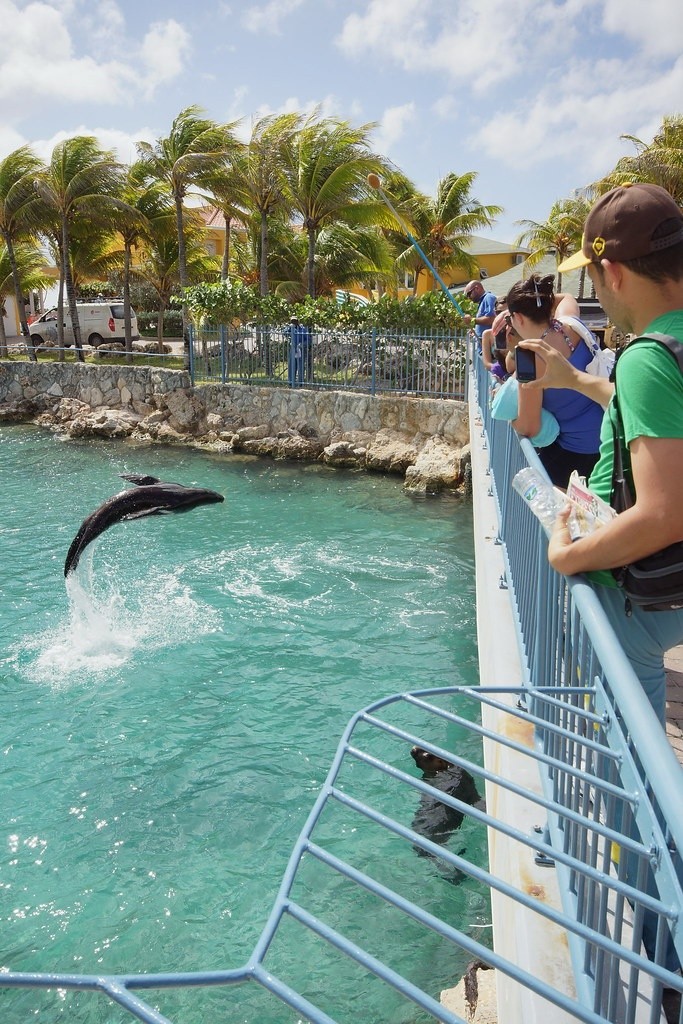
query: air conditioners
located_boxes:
[512,254,525,264]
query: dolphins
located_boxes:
[410,746,481,857]
[63,474,224,580]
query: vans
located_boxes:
[26,302,140,348]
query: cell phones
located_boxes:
[515,346,536,383]
[495,330,507,349]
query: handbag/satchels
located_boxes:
[557,316,618,411]
[608,333,683,616]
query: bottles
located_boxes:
[512,467,582,542]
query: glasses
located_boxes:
[467,288,475,297]
[505,315,513,326]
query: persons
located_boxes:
[549,181,681,974]
[284,316,312,387]
[461,272,612,492]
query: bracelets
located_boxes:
[470,318,476,328]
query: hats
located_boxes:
[557,181,683,273]
